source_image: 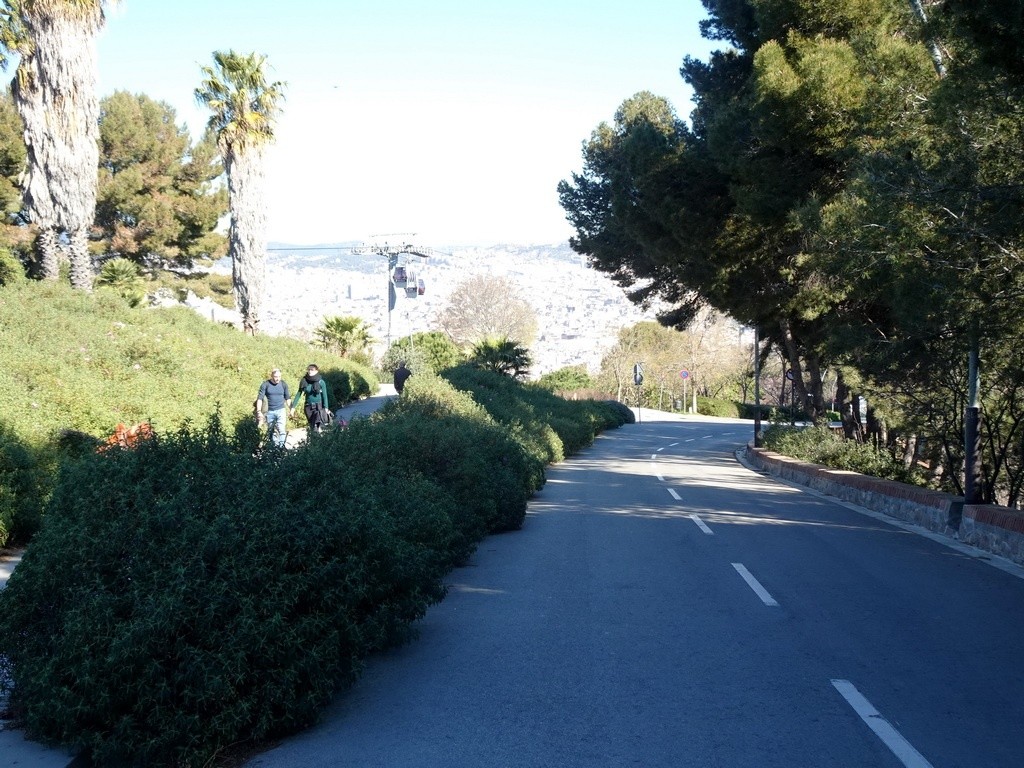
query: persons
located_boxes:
[256,369,295,450]
[286,364,329,443]
[394,360,412,396]
